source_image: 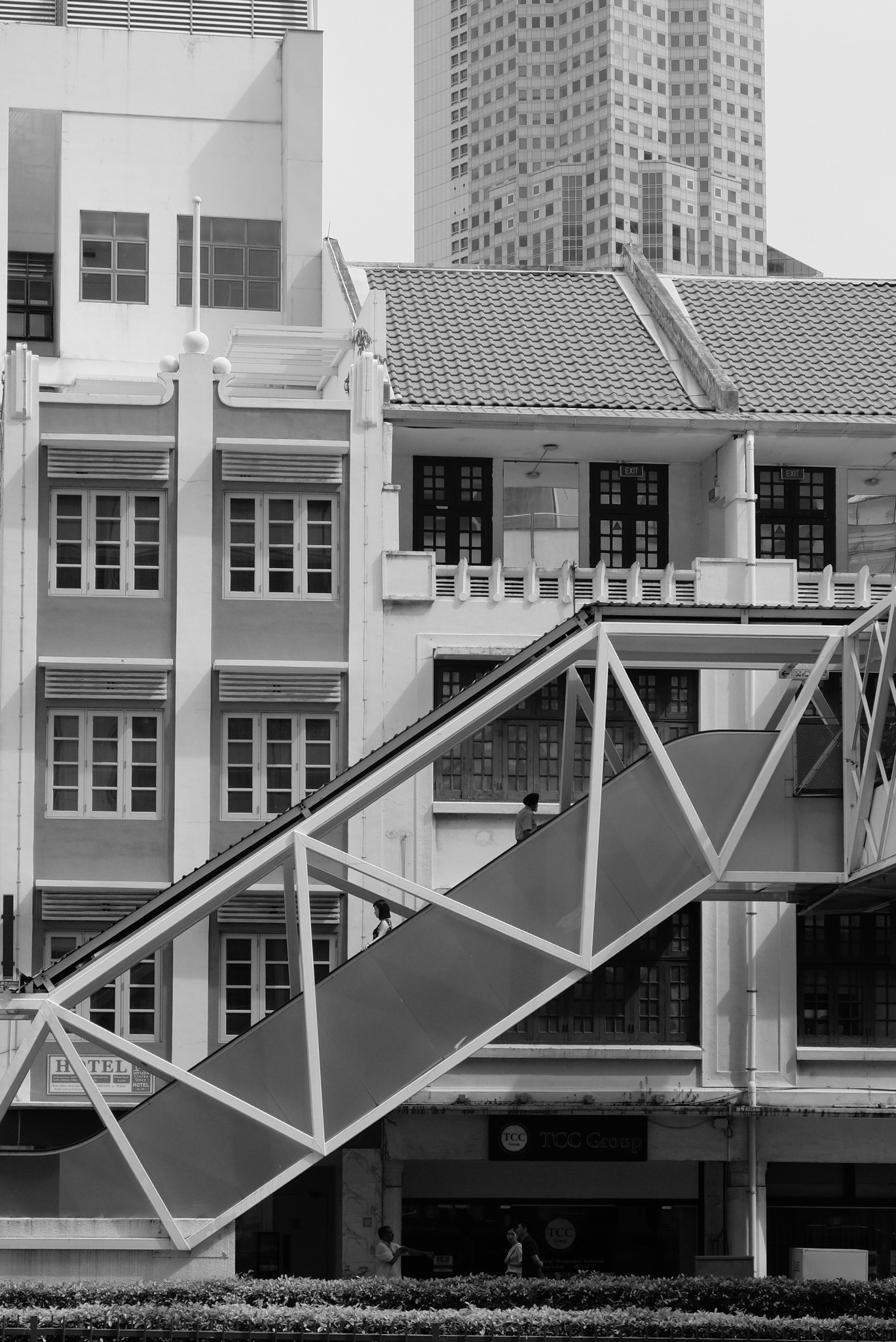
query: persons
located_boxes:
[504,1227,523,1279]
[376,1227,437,1279]
[361,899,392,951]
[515,1221,543,1278]
[515,793,539,846]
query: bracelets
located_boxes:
[365,943,370,945]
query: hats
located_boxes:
[523,793,539,805]
